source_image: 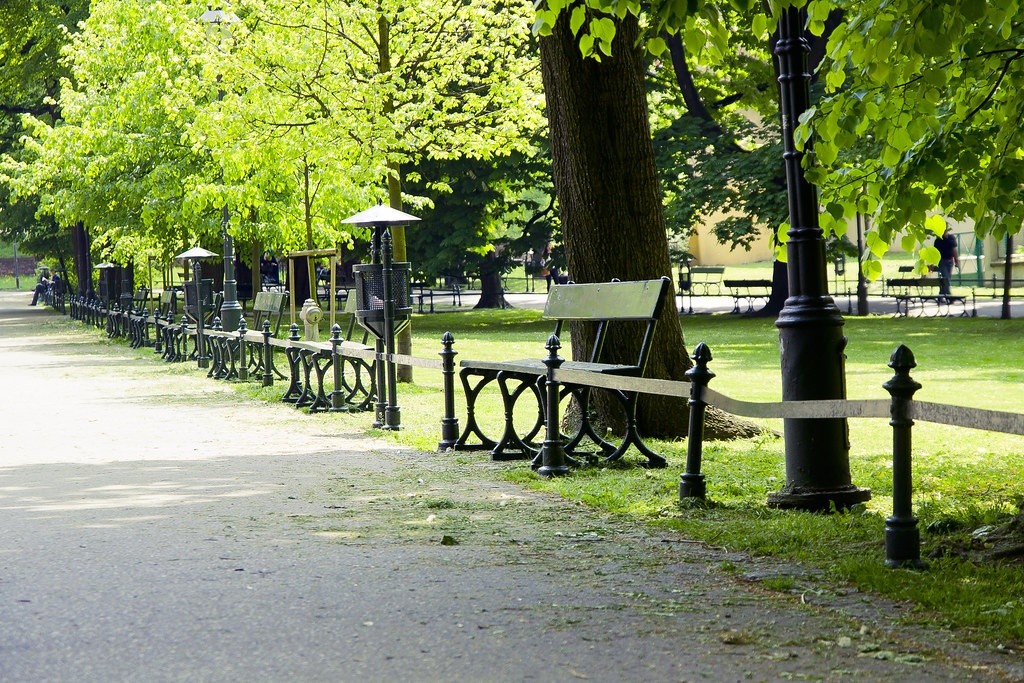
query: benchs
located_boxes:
[886,278,969,318]
[455,276,669,467]
[899,267,942,301]
[689,267,723,295]
[282,289,376,414]
[723,280,772,313]
[215,292,288,383]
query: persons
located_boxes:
[932,220,959,306]
[541,243,559,295]
[180,248,344,306]
[28,270,63,306]
